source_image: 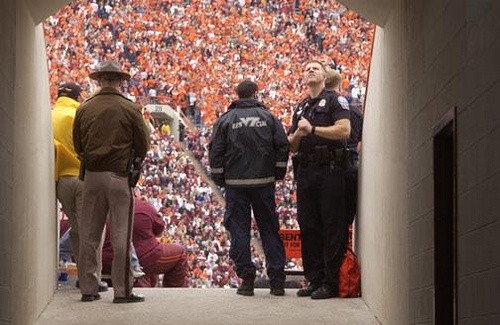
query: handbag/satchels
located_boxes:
[337,247,362,298]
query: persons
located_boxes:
[43,0,377,289]
[51,82,109,292]
[325,69,364,246]
[73,59,151,305]
[287,60,348,299]
[207,79,291,296]
[131,187,187,288]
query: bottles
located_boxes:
[56,256,69,292]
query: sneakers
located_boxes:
[270,281,284,295]
[312,286,338,298]
[297,284,318,296]
[237,280,254,295]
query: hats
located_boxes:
[58,81,87,95]
[89,61,131,81]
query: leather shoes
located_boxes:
[113,294,144,303]
[76,281,108,292]
[81,293,100,301]
